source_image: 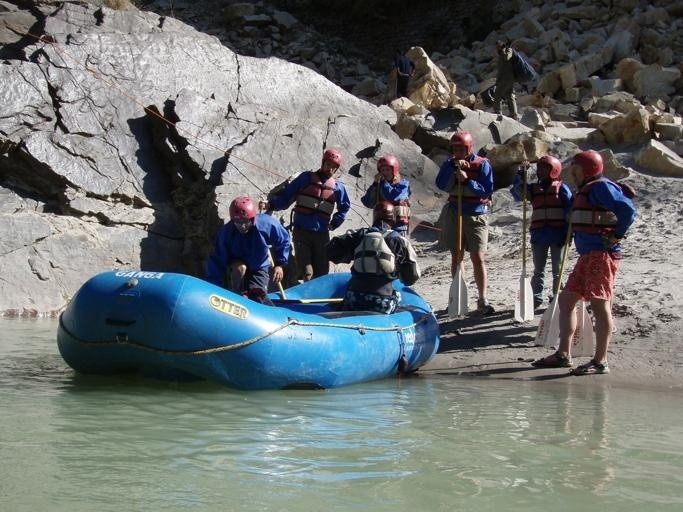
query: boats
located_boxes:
[57,270,440,389]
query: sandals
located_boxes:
[570,360,611,376]
[532,350,573,367]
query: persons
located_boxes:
[508,157,572,310]
[360,155,411,238]
[394,47,415,99]
[436,132,494,315]
[204,197,289,302]
[532,147,635,377]
[494,39,521,121]
[323,204,422,315]
[258,149,349,280]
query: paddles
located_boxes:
[569,298,593,356]
[270,299,342,304]
[533,208,573,347]
[514,164,532,323]
[447,163,468,316]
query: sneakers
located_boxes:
[477,297,496,314]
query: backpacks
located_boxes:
[354,229,395,274]
